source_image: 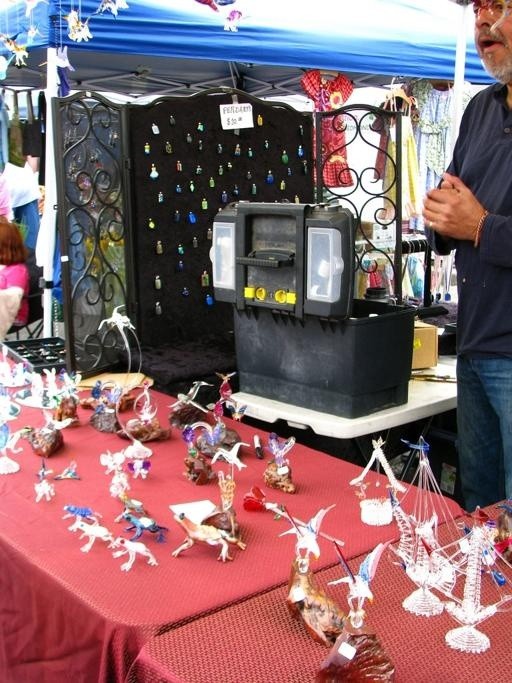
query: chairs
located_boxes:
[6,265,44,340]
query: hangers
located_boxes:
[318,70,339,79]
[383,76,412,111]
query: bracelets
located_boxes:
[472,210,489,249]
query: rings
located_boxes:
[428,221,434,231]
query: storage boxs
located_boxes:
[0,336,66,375]
[412,321,438,370]
[209,199,417,419]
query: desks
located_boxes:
[0,346,468,681]
[219,355,458,479]
[122,500,512,682]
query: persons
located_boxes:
[0,214,28,338]
[7,120,44,265]
[422,0,512,516]
